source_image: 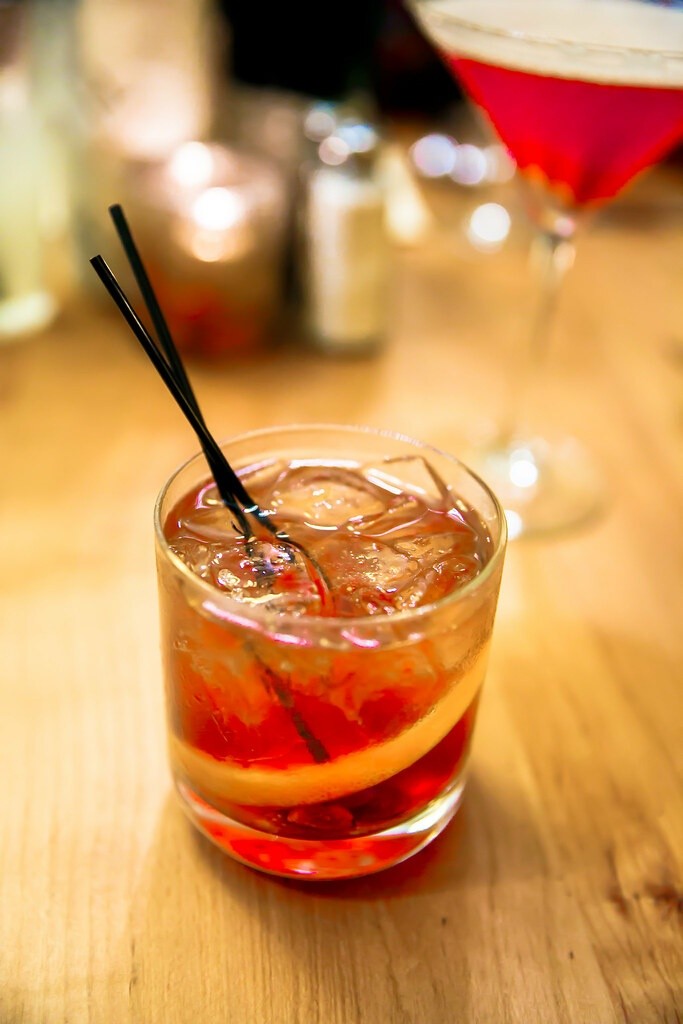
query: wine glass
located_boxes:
[401,0,683,537]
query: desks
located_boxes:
[0,100,683,1024]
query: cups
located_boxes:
[132,148,277,354]
[156,424,507,881]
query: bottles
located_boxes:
[288,101,390,357]
